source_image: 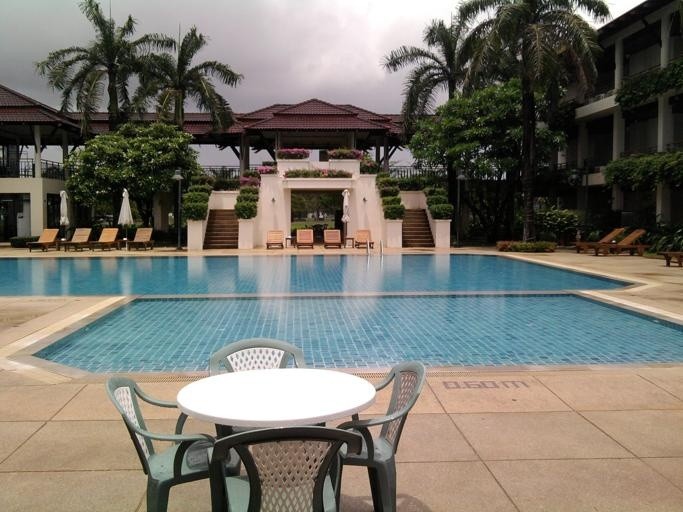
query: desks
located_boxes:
[286,236,296,248]
[175,368,377,430]
[344,238,354,248]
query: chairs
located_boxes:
[126,227,155,251]
[591,228,651,257]
[296,229,314,249]
[209,426,362,512]
[332,361,427,512]
[60,226,93,252]
[26,228,60,252]
[570,227,625,257]
[324,229,341,249]
[266,231,284,249]
[106,376,241,511]
[88,227,121,251]
[656,252,683,267]
[355,229,374,249]
[208,337,305,376]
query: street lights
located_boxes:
[170,167,185,251]
[453,167,468,252]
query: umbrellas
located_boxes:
[59,190,70,252]
[118,187,134,251]
[340,188,352,248]
